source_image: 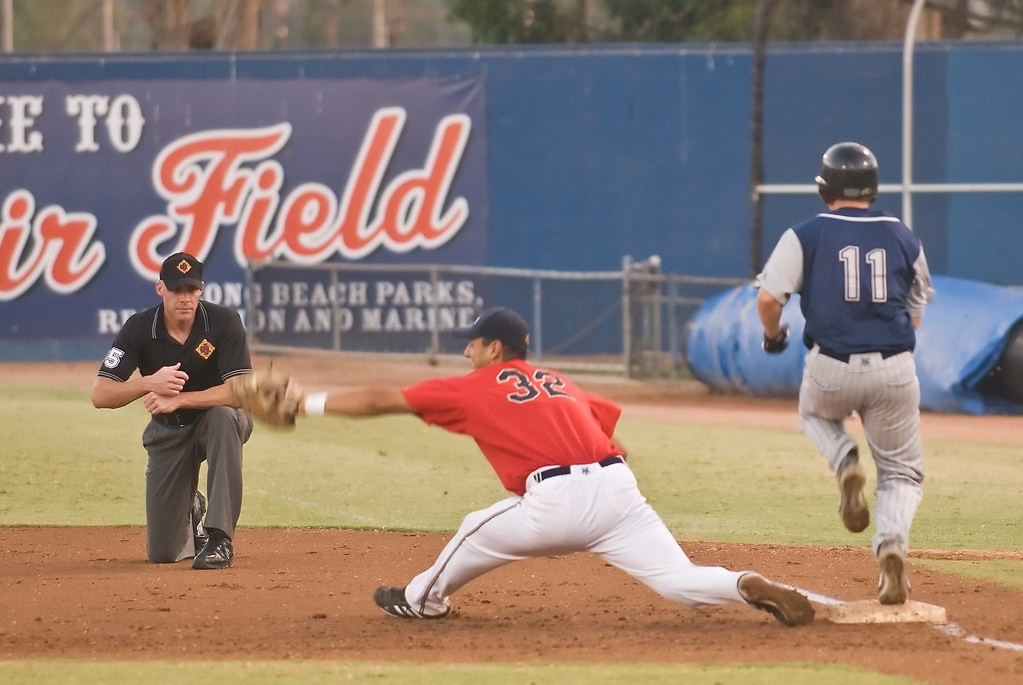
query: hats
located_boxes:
[452,306,528,354]
[160,252,204,292]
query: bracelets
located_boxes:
[305,392,325,416]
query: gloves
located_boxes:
[764,324,789,353]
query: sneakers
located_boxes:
[192,532,232,569]
[736,574,815,624]
[194,491,209,555]
[374,587,453,618]
[877,545,907,603]
[839,459,869,532]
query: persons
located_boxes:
[757,141,934,605]
[92,252,255,569]
[236,306,815,624]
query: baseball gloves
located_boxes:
[230,357,306,431]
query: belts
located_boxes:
[160,415,197,427]
[535,457,623,482]
[810,341,909,363]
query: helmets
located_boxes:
[816,142,879,203]
